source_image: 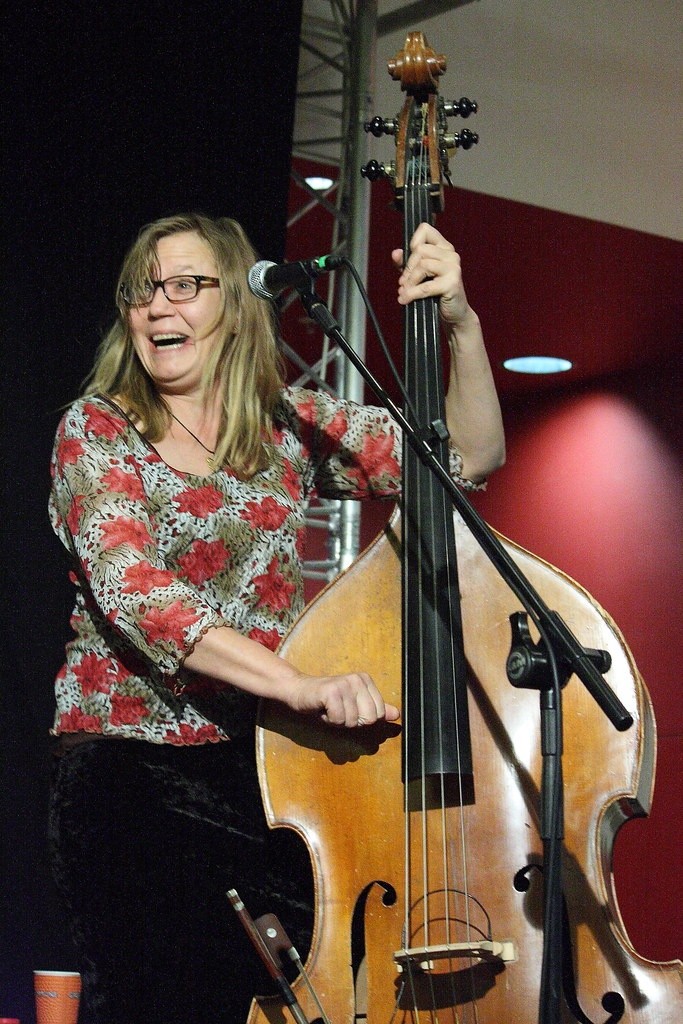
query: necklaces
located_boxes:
[168,409,215,470]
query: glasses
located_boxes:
[121,275,219,305]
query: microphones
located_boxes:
[248,254,345,299]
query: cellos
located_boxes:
[246,28,683,1024]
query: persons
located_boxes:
[48,212,508,1024]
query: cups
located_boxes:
[33,970,81,1024]
